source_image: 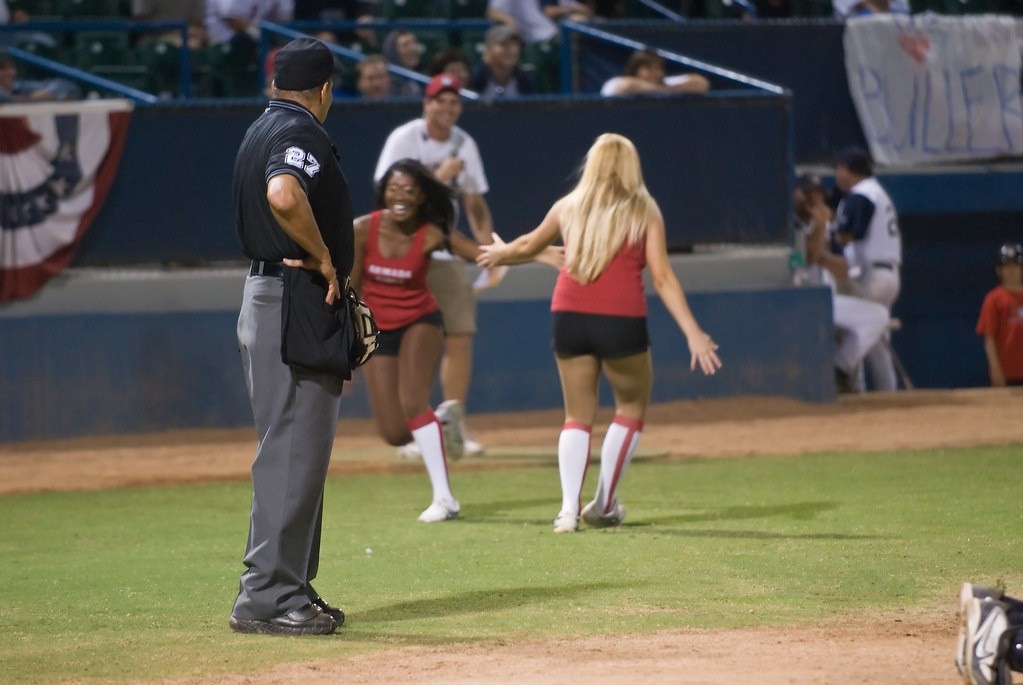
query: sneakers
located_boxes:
[314,596,344,627]
[553,510,580,532]
[438,399,463,462]
[230,602,335,635]
[581,499,626,525]
[954,580,1012,685]
[417,501,460,522]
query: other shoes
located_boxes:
[462,439,483,456]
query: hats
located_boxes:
[486,25,524,43]
[800,173,824,191]
[273,39,335,90]
[426,72,461,96]
[997,243,1023,264]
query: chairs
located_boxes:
[77,30,150,83]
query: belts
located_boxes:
[250,260,285,277]
[871,261,893,270]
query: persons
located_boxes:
[0,0,594,102]
[342,156,569,522]
[832,0,912,24]
[599,48,709,97]
[227,37,356,636]
[720,0,754,18]
[794,146,902,393]
[973,239,1023,387]
[476,134,723,533]
[374,73,508,454]
